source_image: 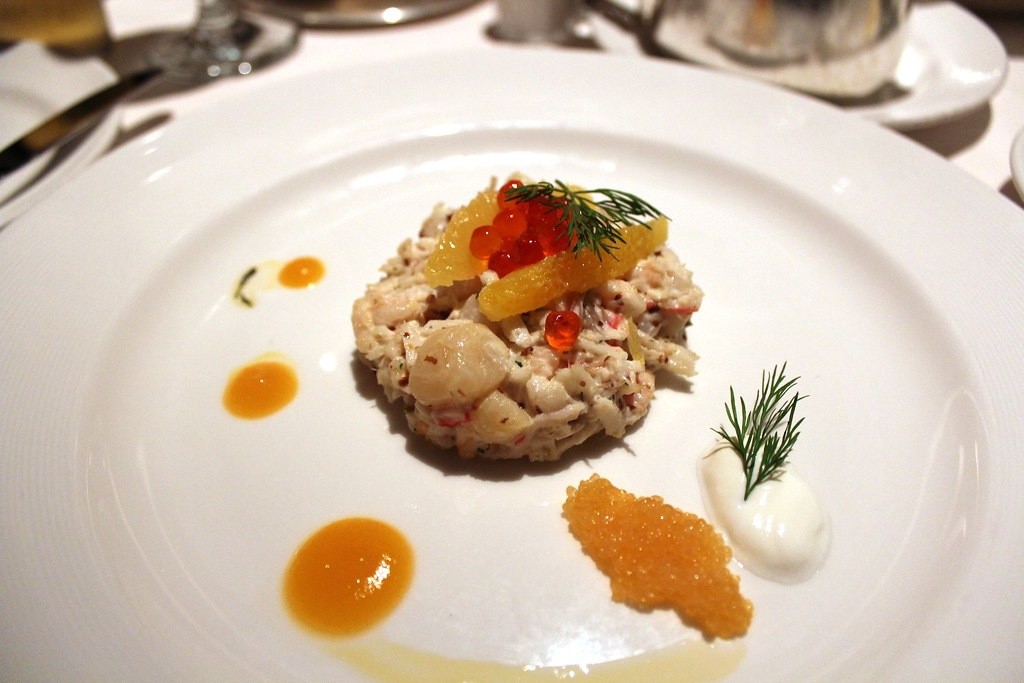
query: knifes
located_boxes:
[0,66,171,179]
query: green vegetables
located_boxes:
[504,180,673,264]
[702,360,811,501]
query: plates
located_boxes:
[0,45,1024,683]
[241,0,490,31]
[588,0,1009,130]
[0,37,120,228]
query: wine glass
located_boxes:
[148,0,299,79]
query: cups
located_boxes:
[582,0,912,104]
[492,0,586,46]
